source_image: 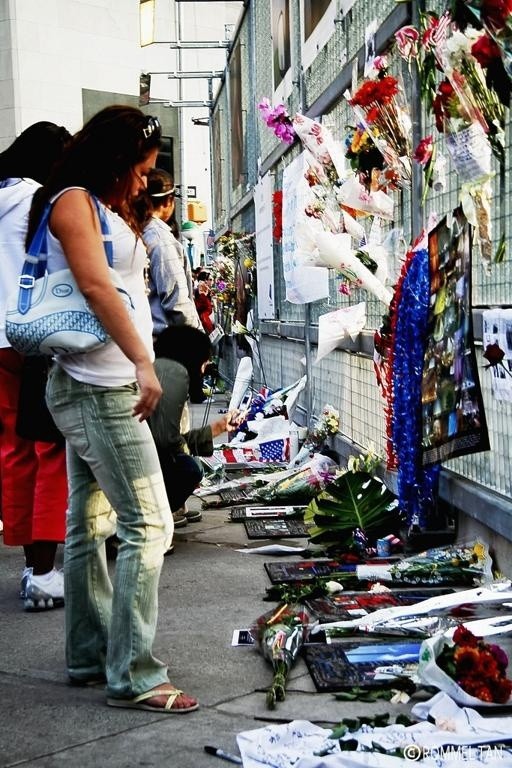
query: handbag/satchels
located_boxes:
[6,188,130,356]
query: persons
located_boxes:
[0,103,244,714]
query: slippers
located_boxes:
[106,681,199,713]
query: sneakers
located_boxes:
[173,509,202,526]
[20,565,67,612]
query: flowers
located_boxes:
[199,389,512,732]
[206,226,269,389]
[254,0,512,315]
